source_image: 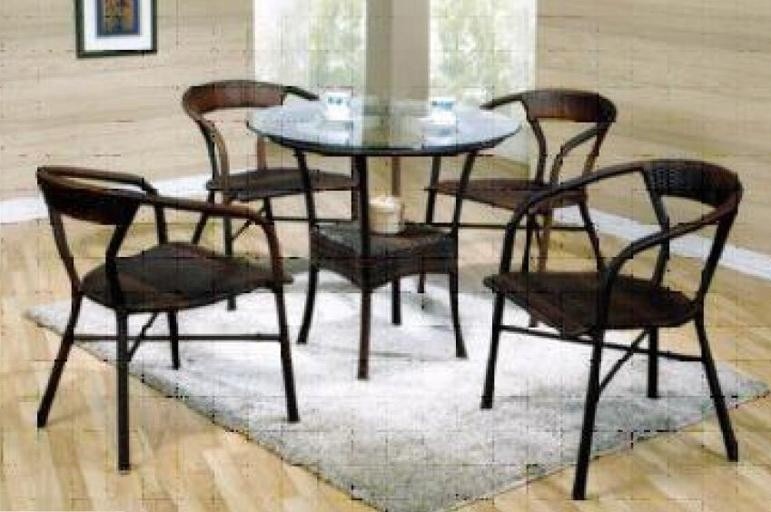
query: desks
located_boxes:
[247,94,522,381]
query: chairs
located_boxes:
[417,90,619,329]
[36,165,300,474]
[481,160,743,499]
[184,80,359,312]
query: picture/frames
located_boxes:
[75,0,158,58]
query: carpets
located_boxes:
[25,271,771,512]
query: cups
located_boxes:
[324,93,354,120]
[430,96,458,127]
